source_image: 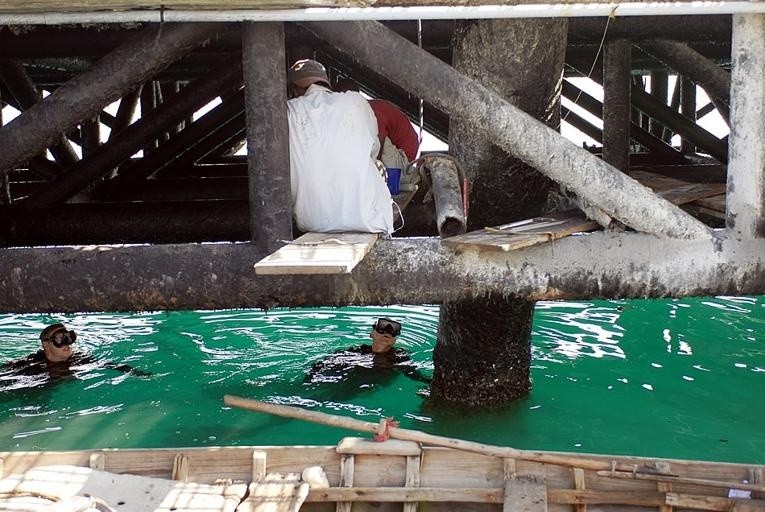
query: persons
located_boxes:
[0,323,152,399]
[304,317,432,404]
[287,58,417,234]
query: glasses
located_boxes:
[373,318,401,337]
[45,328,77,348]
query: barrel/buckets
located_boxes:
[386,168,401,195]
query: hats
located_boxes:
[289,59,332,89]
